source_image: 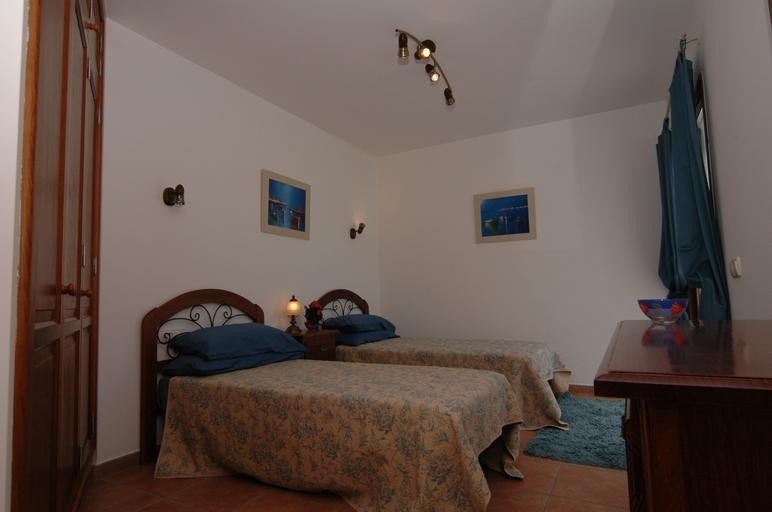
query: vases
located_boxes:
[310,322,319,331]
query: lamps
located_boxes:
[394,29,456,106]
[163,184,186,207]
[350,223,365,239]
[285,296,302,336]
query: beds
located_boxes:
[318,289,570,431]
[141,289,524,512]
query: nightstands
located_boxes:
[294,329,340,361]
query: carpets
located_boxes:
[524,391,626,471]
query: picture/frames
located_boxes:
[261,168,311,240]
[474,187,537,243]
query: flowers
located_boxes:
[305,301,323,329]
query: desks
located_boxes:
[594,320,772,512]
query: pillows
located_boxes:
[324,315,395,332]
[336,330,401,346]
[168,323,306,359]
[158,352,306,377]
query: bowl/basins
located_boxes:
[636,299,690,324]
[640,321,691,350]
